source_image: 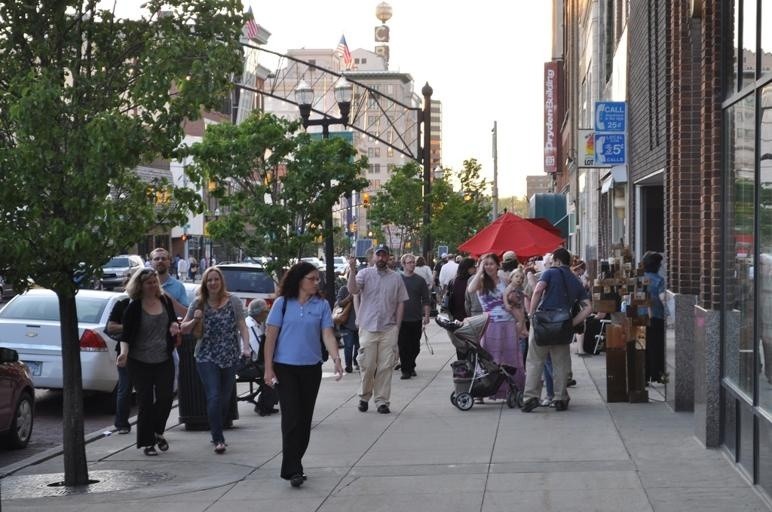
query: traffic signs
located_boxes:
[594,101,626,165]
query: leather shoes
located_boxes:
[411,371,416,376]
[521,398,539,412]
[255,406,267,417]
[567,378,577,387]
[290,474,304,488]
[400,374,411,379]
[555,400,566,411]
[271,409,279,413]
[358,400,368,412]
[302,473,307,480]
[378,405,390,414]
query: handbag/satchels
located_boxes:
[193,297,204,338]
[532,267,574,346]
[258,296,287,362]
[331,301,351,324]
[164,295,182,347]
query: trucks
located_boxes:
[356,239,373,264]
[438,246,448,263]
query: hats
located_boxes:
[375,244,389,255]
[503,251,517,263]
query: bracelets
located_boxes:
[334,357,341,363]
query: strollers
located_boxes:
[435,314,524,410]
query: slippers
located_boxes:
[540,397,551,407]
[214,442,226,454]
[473,398,483,404]
[144,447,158,456]
[549,400,555,408]
[157,438,169,451]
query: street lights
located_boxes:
[294,73,354,312]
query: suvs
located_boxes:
[197,263,278,311]
[93,255,144,288]
[334,257,350,280]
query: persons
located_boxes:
[524,255,554,407]
[448,258,477,361]
[499,251,587,280]
[338,270,361,373]
[413,256,434,316]
[149,248,188,321]
[522,248,592,412]
[644,253,666,386]
[348,245,408,413]
[466,255,484,317]
[502,269,530,339]
[181,268,250,453]
[117,268,179,455]
[433,253,448,305]
[237,299,279,415]
[108,299,133,434]
[264,262,344,486]
[145,258,149,268]
[439,254,459,290]
[759,254,772,389]
[399,254,430,379]
[388,254,403,275]
[169,254,216,282]
[468,253,526,403]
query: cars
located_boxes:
[0,288,179,414]
[0,347,35,449]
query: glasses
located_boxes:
[406,262,416,265]
[305,276,321,282]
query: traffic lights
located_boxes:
[180,234,192,241]
[363,193,369,208]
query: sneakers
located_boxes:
[118,428,129,434]
[345,367,352,373]
[354,365,359,370]
